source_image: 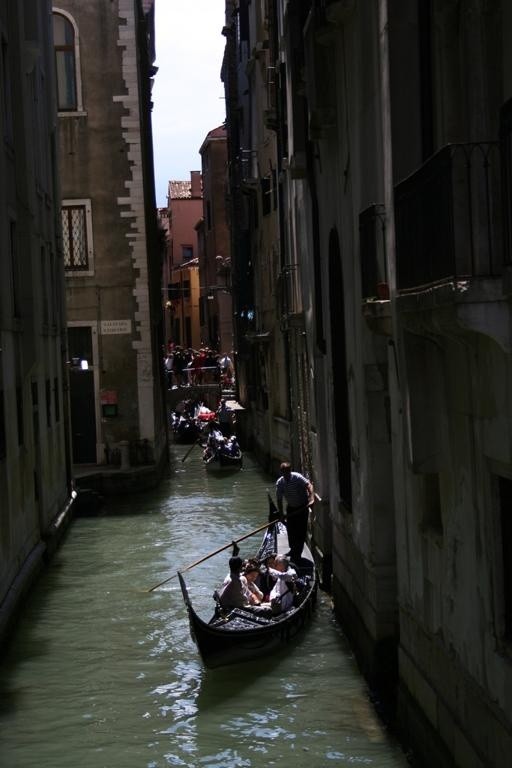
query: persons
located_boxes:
[275,461,314,561]
[216,553,299,621]
[162,343,241,463]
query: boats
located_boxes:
[171,400,244,477]
[173,490,318,672]
